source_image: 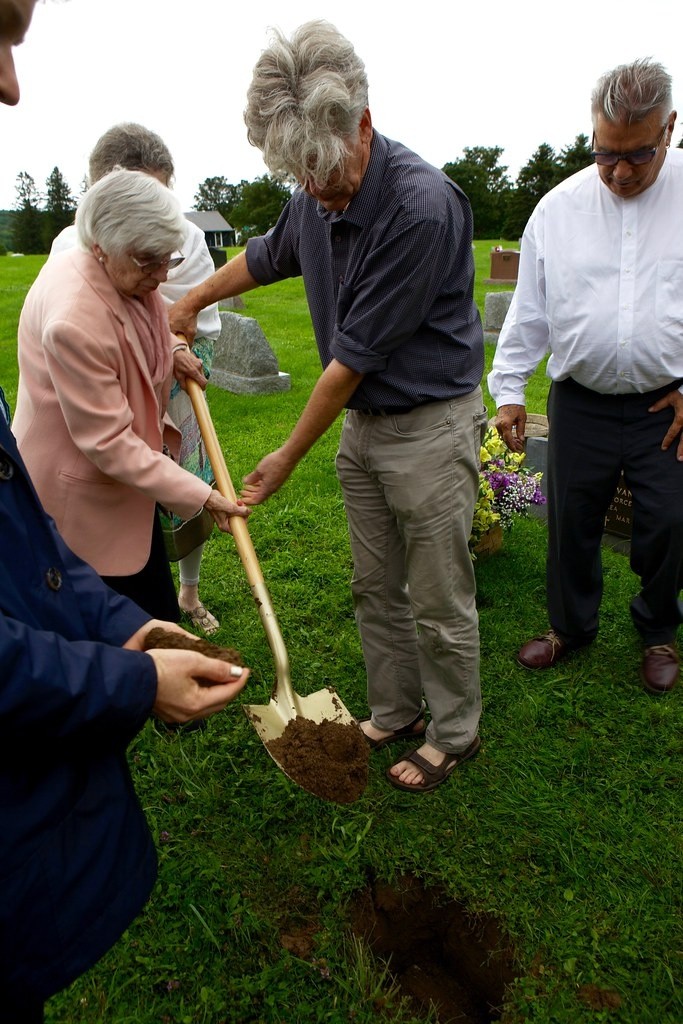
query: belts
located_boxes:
[357,398,442,416]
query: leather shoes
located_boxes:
[517,626,594,671]
[638,641,679,693]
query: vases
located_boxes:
[472,524,503,560]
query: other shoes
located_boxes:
[151,715,206,733]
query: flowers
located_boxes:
[467,426,547,560]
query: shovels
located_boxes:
[176,332,371,804]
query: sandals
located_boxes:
[385,734,480,793]
[357,709,427,751]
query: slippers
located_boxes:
[182,602,219,637]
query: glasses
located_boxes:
[590,116,669,166]
[125,249,186,275]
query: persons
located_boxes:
[11,169,253,629]
[486,65,683,694]
[89,122,222,637]
[166,21,484,791]
[0,0,250,1024]
